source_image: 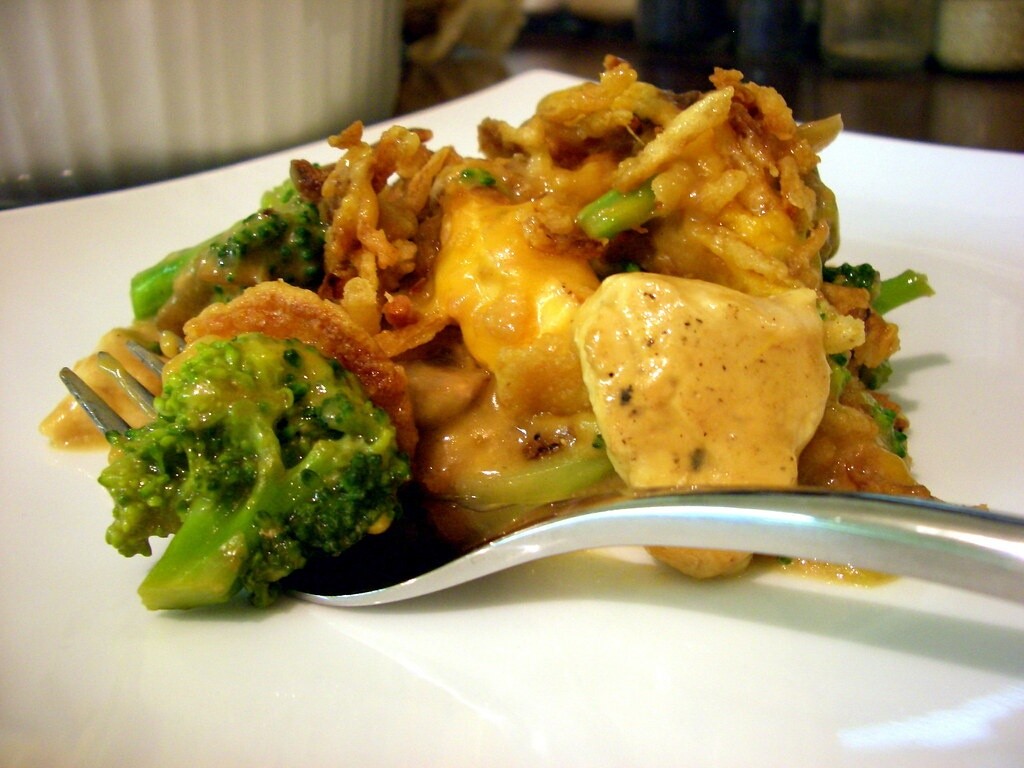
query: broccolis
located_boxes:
[95,165,938,613]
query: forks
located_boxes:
[61,333,1024,607]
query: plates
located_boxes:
[1,64,1021,765]
